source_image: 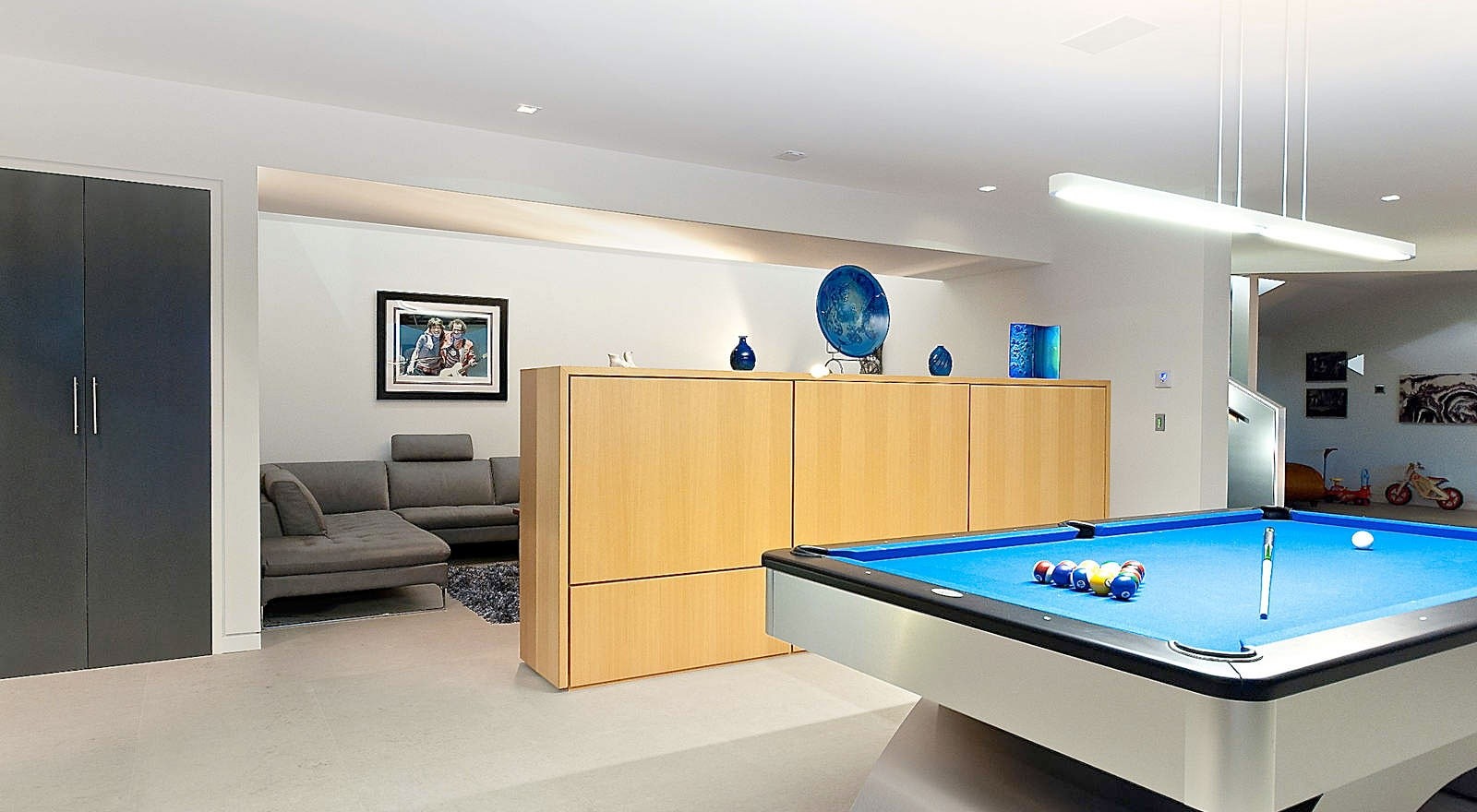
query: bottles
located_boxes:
[928,345,953,376]
[730,335,756,371]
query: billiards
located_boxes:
[1032,559,1146,601]
[1351,530,1374,549]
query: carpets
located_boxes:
[442,560,521,625]
[440,562,520,628]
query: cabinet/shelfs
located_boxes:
[517,367,1119,692]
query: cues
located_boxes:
[1258,526,1276,621]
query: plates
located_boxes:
[816,265,890,358]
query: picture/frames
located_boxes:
[1397,371,1477,426]
[369,285,513,409]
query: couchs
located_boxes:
[259,430,518,623]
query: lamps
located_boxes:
[1040,0,1420,273]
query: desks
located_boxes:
[760,503,1477,812]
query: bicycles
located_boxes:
[1385,460,1466,510]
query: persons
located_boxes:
[405,317,478,376]
